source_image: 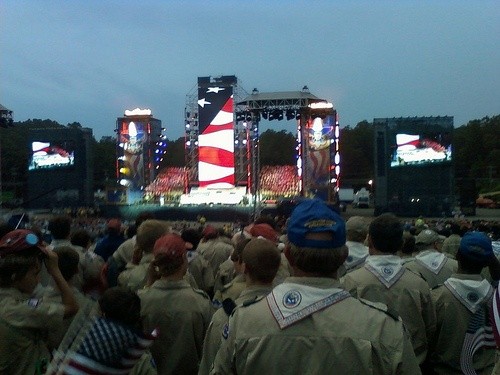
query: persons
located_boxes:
[32,142,71,166]
[127,122,137,146]
[0,164,500,375]
[310,117,324,143]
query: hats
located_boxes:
[287,200,347,248]
[346,216,370,234]
[204,226,218,239]
[154,234,187,261]
[0,229,48,256]
[108,219,121,229]
[250,223,277,244]
[415,228,439,245]
[460,232,492,260]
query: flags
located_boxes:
[45,308,158,375]
